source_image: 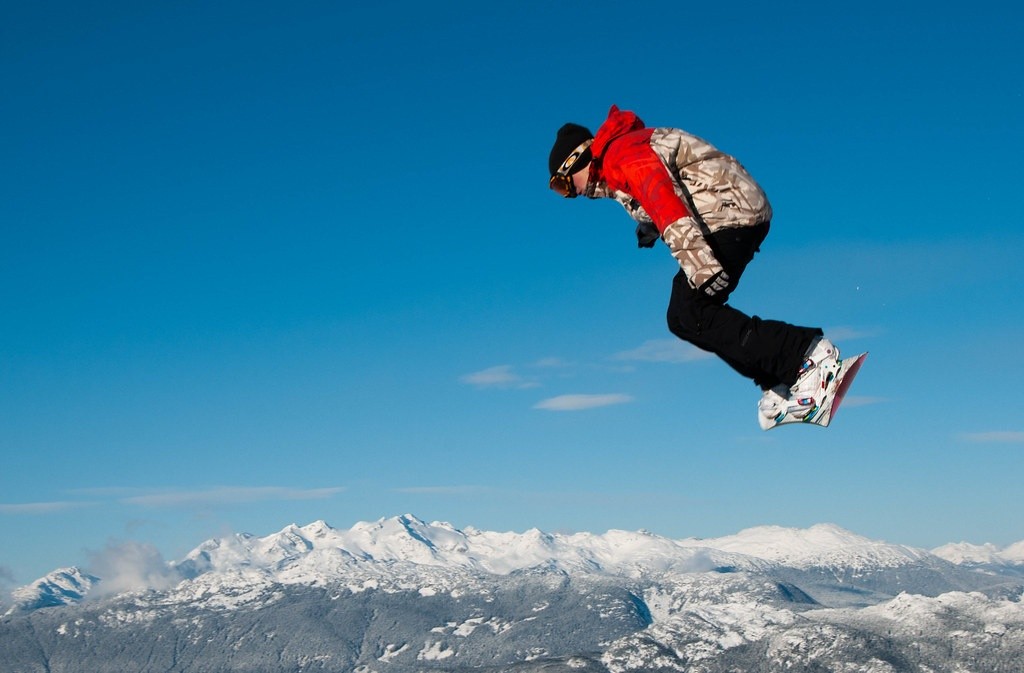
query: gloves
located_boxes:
[702,271,729,295]
[635,222,659,248]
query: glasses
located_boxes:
[549,173,570,198]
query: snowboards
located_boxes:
[757,349,870,432]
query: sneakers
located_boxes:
[758,383,789,431]
[787,336,839,418]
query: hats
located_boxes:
[549,123,593,176]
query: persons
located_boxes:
[548,105,840,419]
